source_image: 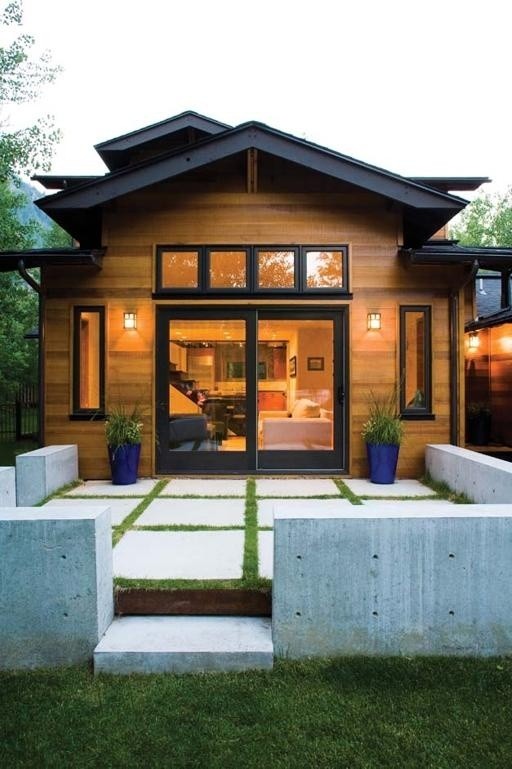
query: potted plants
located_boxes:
[87,384,153,485]
[359,379,409,484]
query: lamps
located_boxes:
[123,312,136,331]
[367,312,382,332]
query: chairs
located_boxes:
[202,402,232,440]
[258,398,333,450]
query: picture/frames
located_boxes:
[288,356,296,377]
[307,357,324,371]
